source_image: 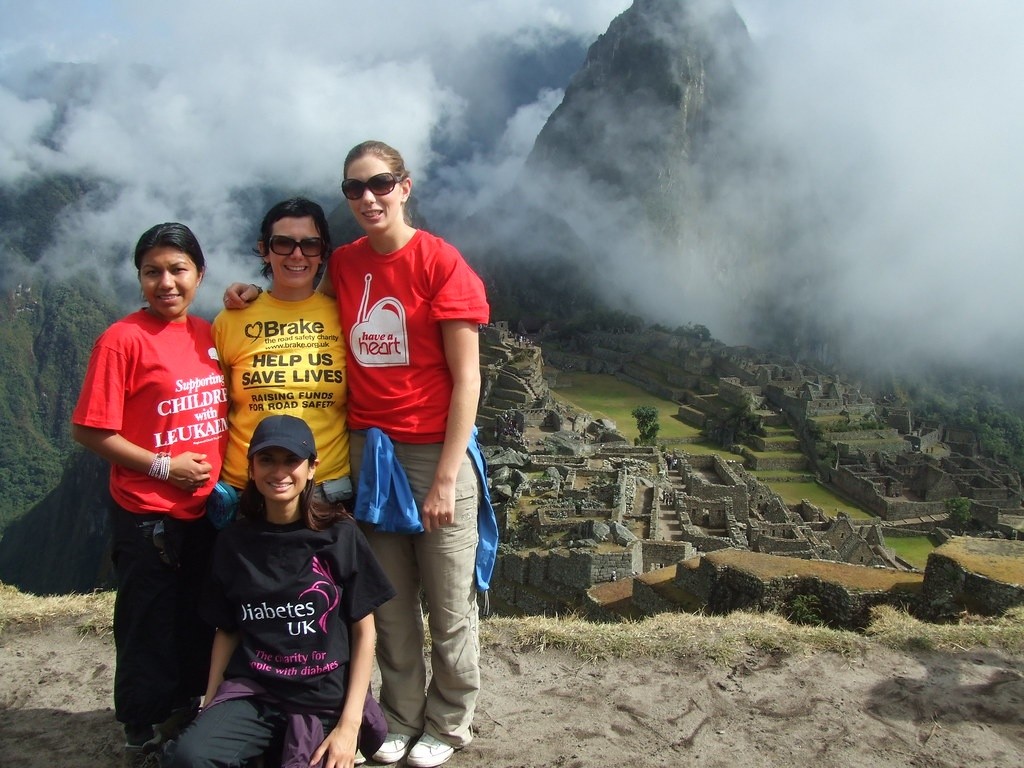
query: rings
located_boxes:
[224,297,229,302]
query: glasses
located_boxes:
[269,234,325,258]
[341,172,407,201]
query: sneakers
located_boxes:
[407,732,455,768]
[373,731,412,763]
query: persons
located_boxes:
[224,140,489,768]
[211,197,351,507]
[72,223,232,753]
[162,415,399,768]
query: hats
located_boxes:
[247,414,317,460]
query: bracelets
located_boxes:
[250,284,263,293]
[148,452,170,480]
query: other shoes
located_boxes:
[124,731,163,768]
